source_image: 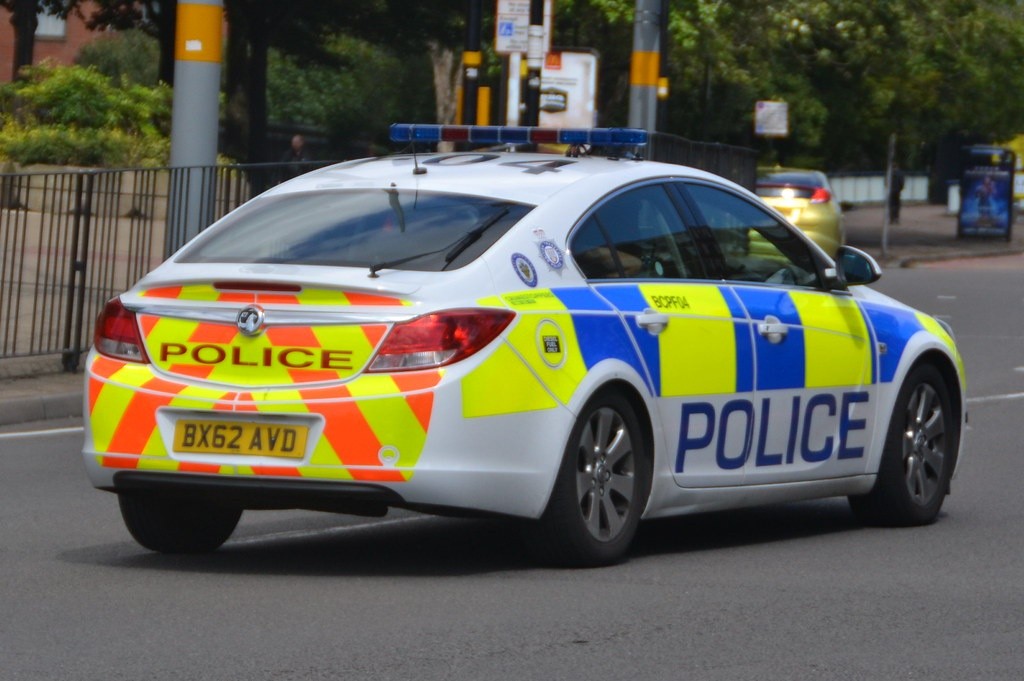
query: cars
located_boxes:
[743,164,850,280]
[81,123,968,568]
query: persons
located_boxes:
[975,176,998,224]
[274,134,314,182]
